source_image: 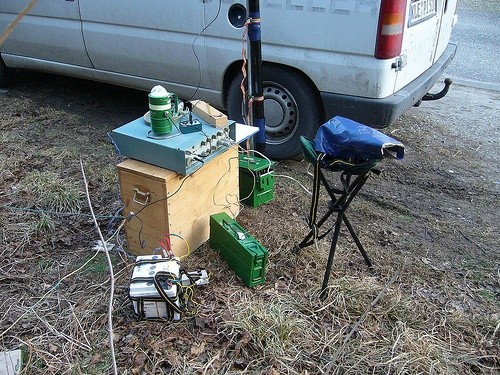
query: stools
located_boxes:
[292,135,377,302]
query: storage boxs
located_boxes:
[116,144,240,260]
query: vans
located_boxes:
[0,0,458,161]
